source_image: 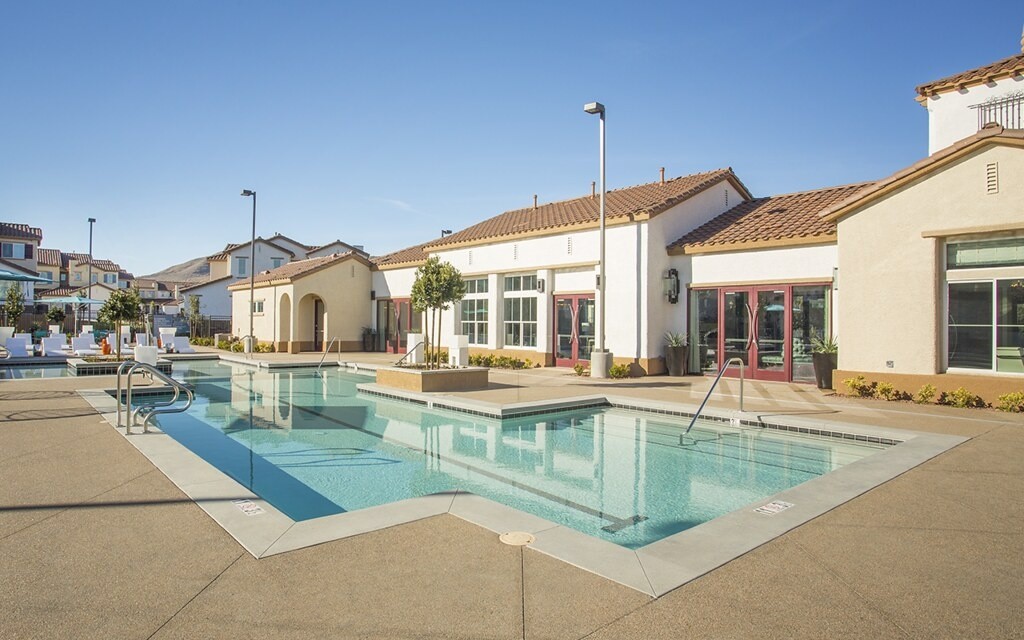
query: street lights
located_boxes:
[438,229,455,240]
[85,217,96,325]
[581,101,615,379]
[239,188,258,354]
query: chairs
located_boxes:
[0,325,195,356]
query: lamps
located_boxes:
[665,268,680,304]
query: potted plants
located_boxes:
[662,331,692,376]
[361,326,375,351]
[808,331,839,389]
